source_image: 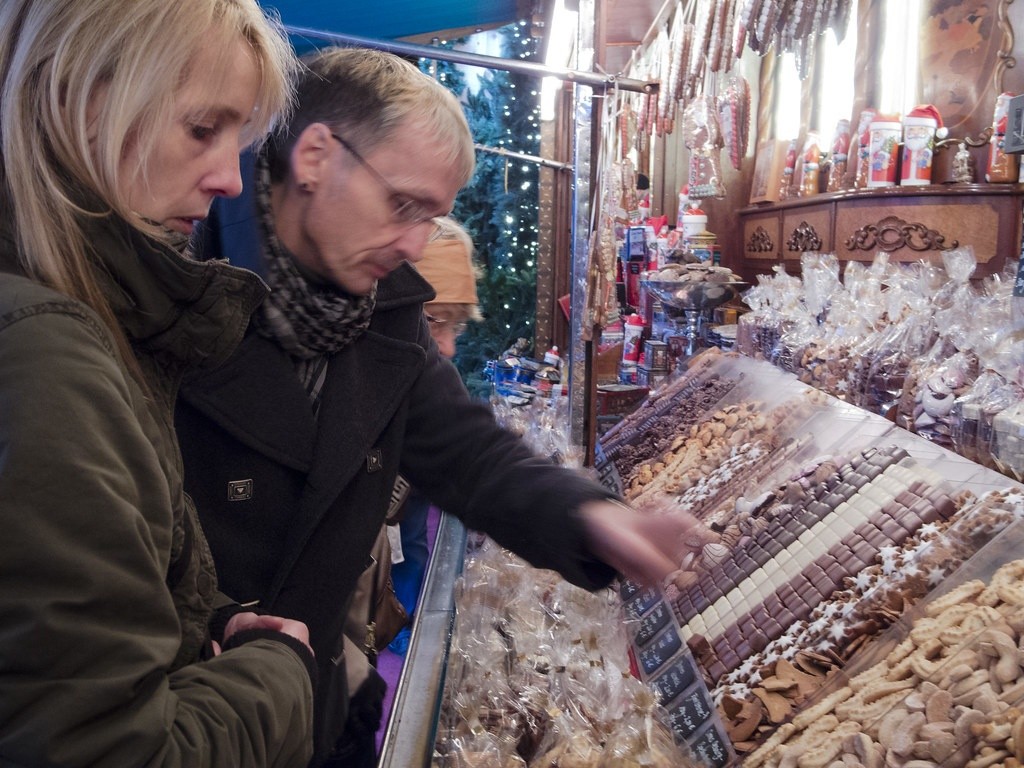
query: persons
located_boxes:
[0,0,710,767]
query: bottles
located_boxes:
[676,184,702,231]
[622,313,645,364]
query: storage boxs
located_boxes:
[713,307,736,325]
[596,335,689,437]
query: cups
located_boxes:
[900,116,936,186]
[868,115,902,187]
[682,214,707,240]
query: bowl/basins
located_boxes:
[637,279,751,310]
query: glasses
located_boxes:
[423,309,468,336]
[331,133,448,243]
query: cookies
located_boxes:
[432,305,1024,768]
